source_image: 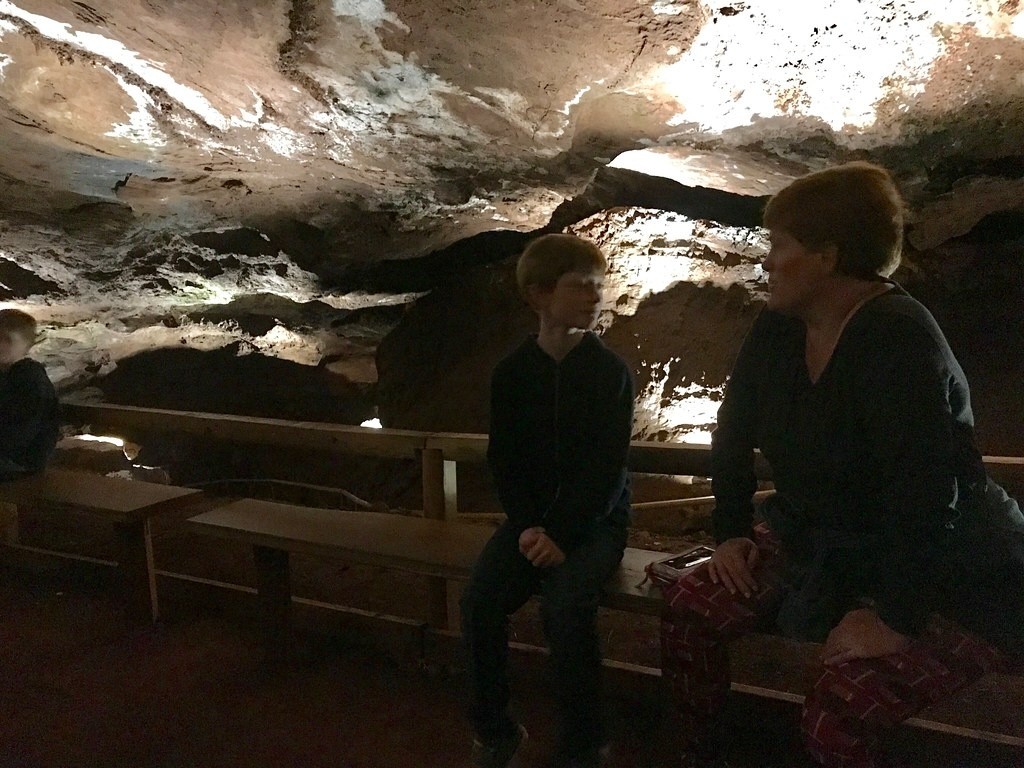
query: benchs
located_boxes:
[186,498,1023,768]
[1,469,204,622]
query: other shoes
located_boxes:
[468,723,528,768]
[555,743,611,768]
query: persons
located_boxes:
[659,160,1024,768]
[0,309,60,482]
[460,233,635,768]
